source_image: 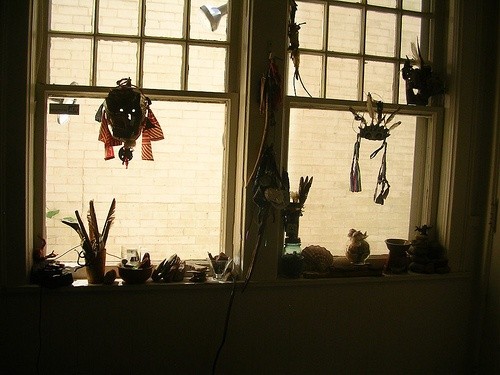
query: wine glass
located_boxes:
[211,260,227,281]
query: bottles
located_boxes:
[280,238,305,280]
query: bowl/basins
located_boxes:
[385,238,412,253]
[118,266,153,284]
